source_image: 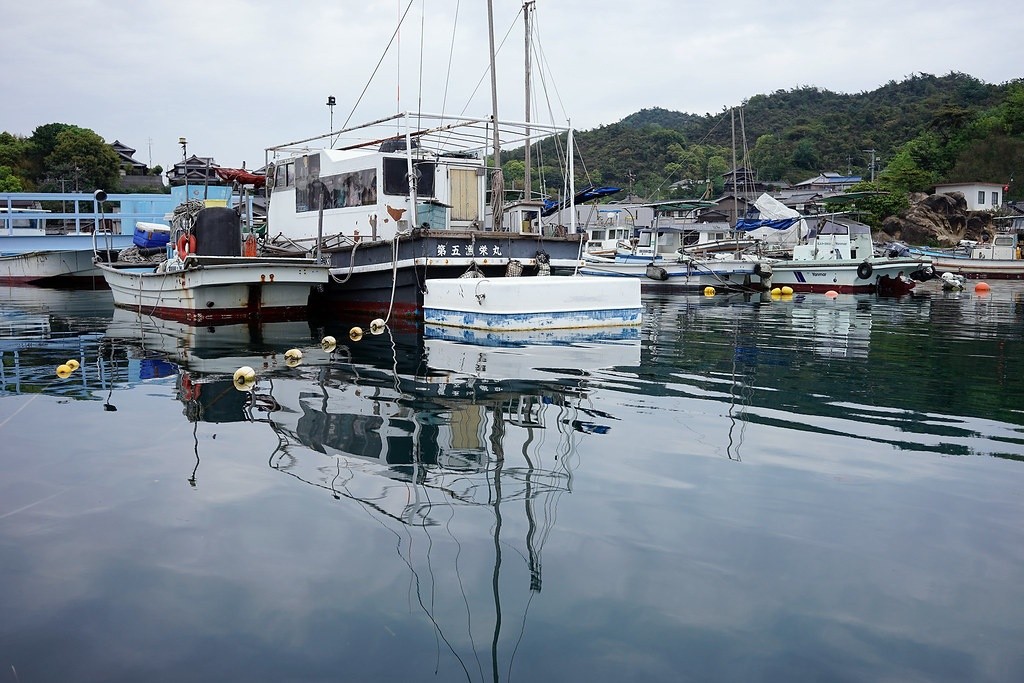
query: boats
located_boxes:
[0,0,1024,335]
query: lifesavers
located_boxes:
[857,262,872,279]
[246,237,256,256]
[177,233,196,260]
